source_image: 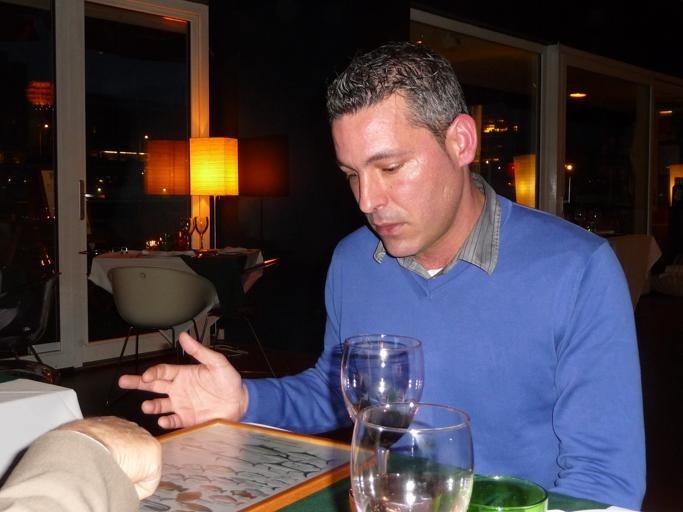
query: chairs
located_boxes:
[0,271,62,362]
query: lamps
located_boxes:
[190,137,239,250]
[144,139,191,198]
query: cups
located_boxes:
[342,333,549,511]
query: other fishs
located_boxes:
[140,435,339,511]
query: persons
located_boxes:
[118,41,648,511]
[5,184,49,329]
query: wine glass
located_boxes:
[176,216,209,251]
